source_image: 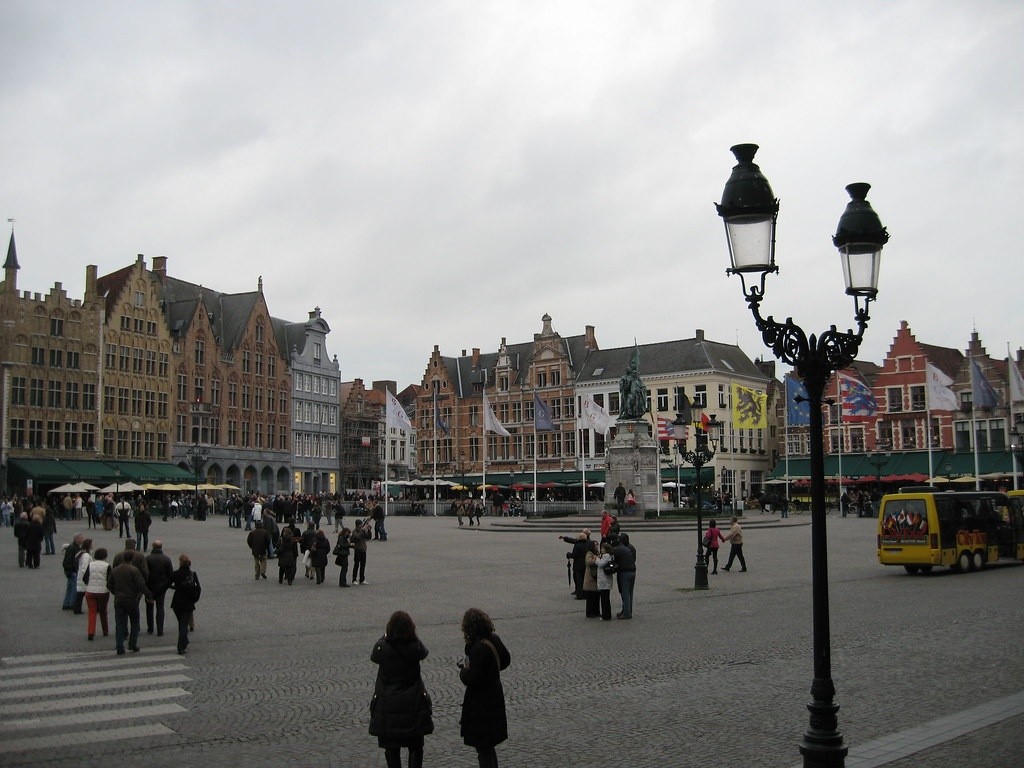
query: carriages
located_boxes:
[754,492,837,519]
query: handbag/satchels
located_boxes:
[188,572,197,600]
[702,529,712,547]
[310,541,316,557]
[332,545,341,555]
[600,553,618,575]
[591,564,597,578]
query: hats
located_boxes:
[355,520,363,526]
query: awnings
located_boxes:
[424,472,606,486]
[765,450,945,480]
[935,451,1024,478]
[9,457,203,484]
[660,468,715,483]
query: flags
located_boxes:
[926,350,1024,413]
[387,389,554,434]
[580,394,610,436]
[881,511,928,534]
[657,372,878,440]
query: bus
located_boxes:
[875,486,1020,573]
[1008,490,1024,561]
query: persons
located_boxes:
[368,611,434,768]
[206,493,254,531]
[134,503,152,552]
[452,494,526,527]
[254,487,381,533]
[60,532,203,653]
[410,500,427,517]
[558,480,639,621]
[246,508,331,586]
[85,492,117,531]
[160,493,208,522]
[841,490,873,518]
[52,493,83,521]
[114,497,132,538]
[455,608,511,768]
[712,486,790,519]
[0,493,57,570]
[331,497,387,587]
[705,515,747,574]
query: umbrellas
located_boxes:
[662,481,687,500]
[762,471,984,493]
[450,482,606,501]
[47,481,242,501]
[382,478,460,499]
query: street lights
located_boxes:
[113,465,120,504]
[671,396,722,589]
[186,441,208,520]
[712,143,890,767]
[459,451,466,500]
[509,466,515,500]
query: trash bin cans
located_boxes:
[865,502,872,510]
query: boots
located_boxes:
[711,561,717,574]
[705,561,708,570]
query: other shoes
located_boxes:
[585,610,632,621]
[62,604,194,655]
[722,566,729,571]
[254,570,369,587]
[739,569,746,572]
